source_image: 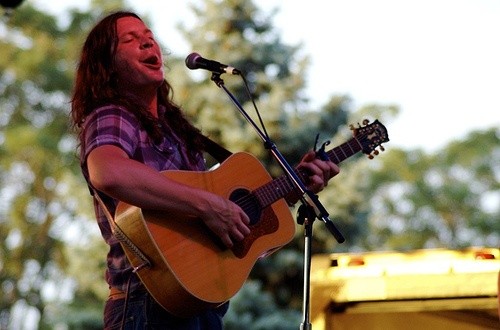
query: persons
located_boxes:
[72,11,340,330]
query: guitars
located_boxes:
[112,118,391,317]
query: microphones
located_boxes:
[185,53,240,75]
